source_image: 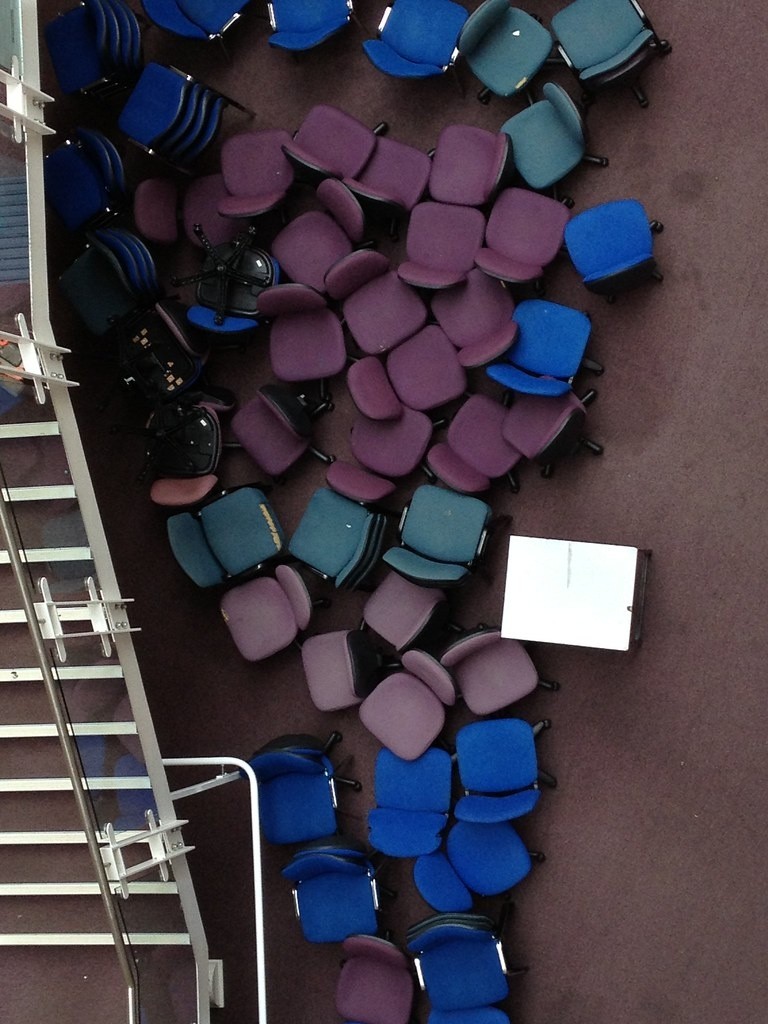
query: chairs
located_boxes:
[37,0,676,1024]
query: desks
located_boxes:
[500,533,653,652]
